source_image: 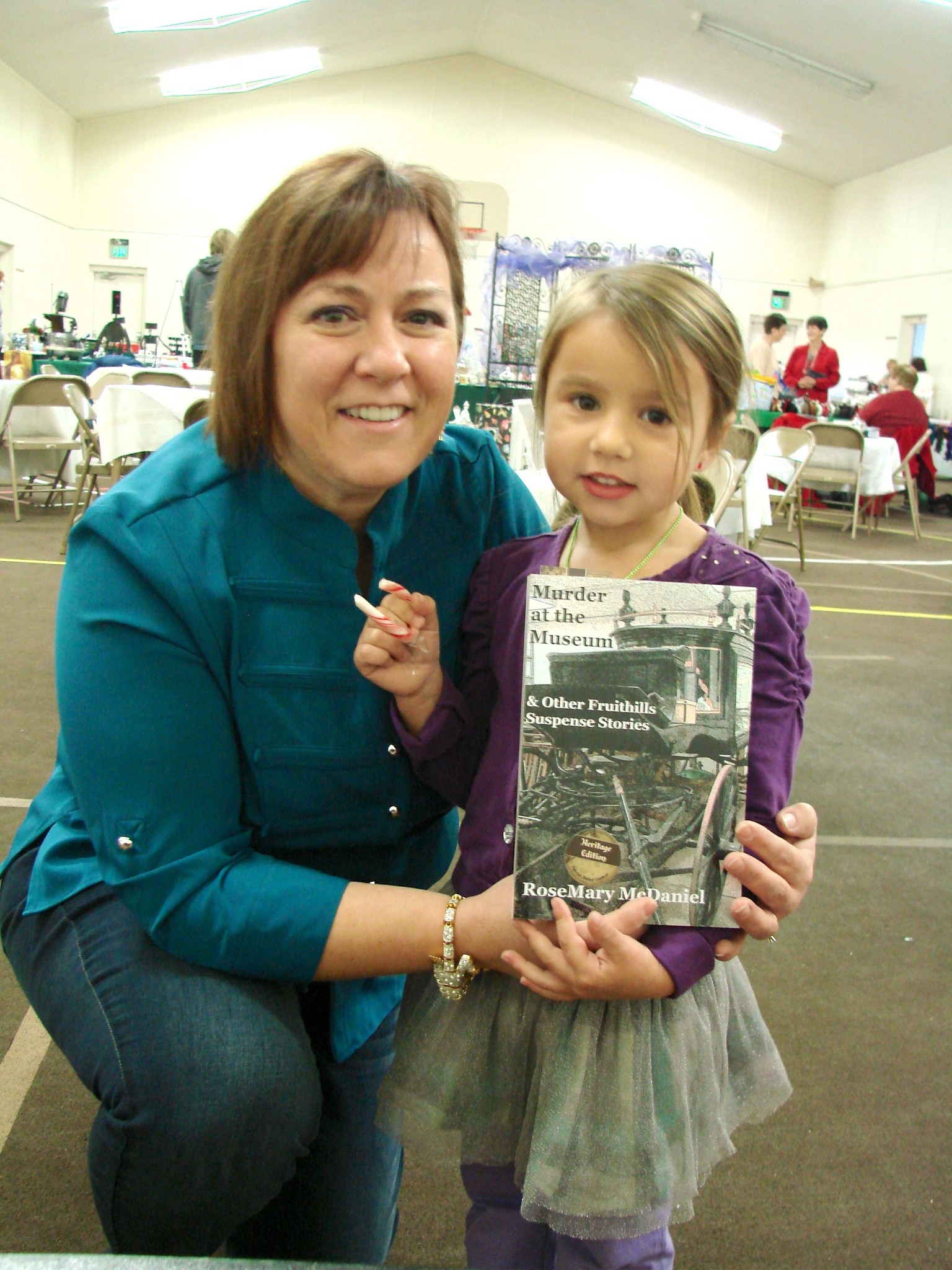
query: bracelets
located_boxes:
[427,893,490,1000]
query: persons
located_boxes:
[0,150,818,1270]
[353,264,811,1270]
[878,360,899,388]
[908,356,934,417]
[747,312,787,398]
[815,367,937,515]
[783,316,840,405]
[182,229,237,370]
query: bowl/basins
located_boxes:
[865,426,882,439]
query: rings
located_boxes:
[755,912,779,944]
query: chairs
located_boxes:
[2,353,214,556]
[680,423,933,573]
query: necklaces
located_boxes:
[563,506,684,581]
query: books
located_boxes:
[512,565,757,931]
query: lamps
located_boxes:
[693,13,875,93]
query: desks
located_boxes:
[515,457,773,552]
[31,358,92,374]
[771,413,937,518]
[444,383,535,463]
[87,365,214,391]
[61,384,213,556]
[1,379,85,483]
